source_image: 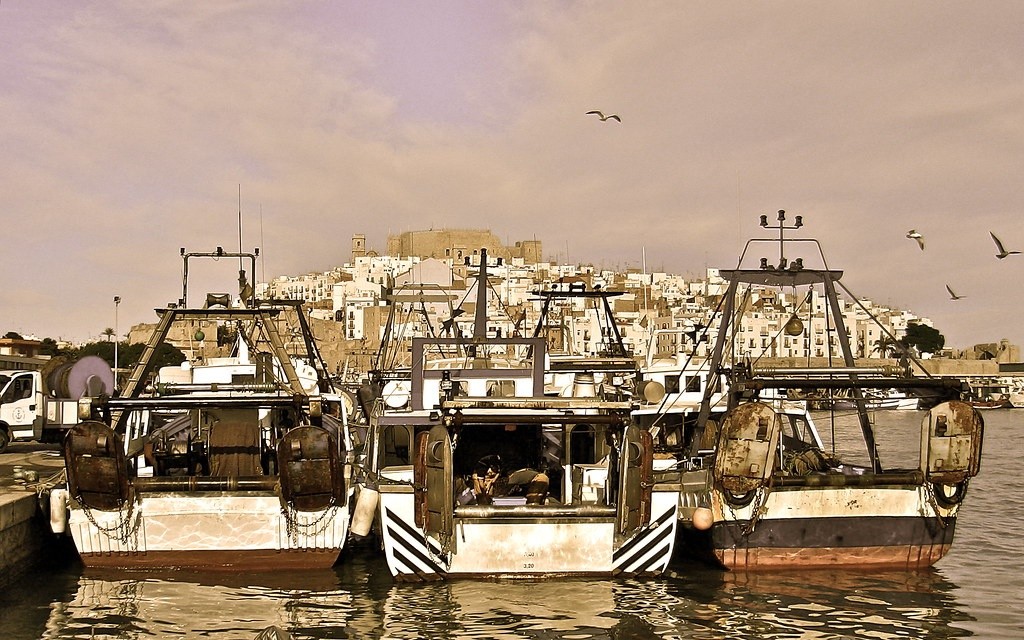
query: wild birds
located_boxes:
[585,110,622,124]
[988,230,1024,261]
[945,283,968,302]
[904,227,926,251]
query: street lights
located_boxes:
[112,295,127,392]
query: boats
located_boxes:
[234,352,1024,415]
[47,248,364,572]
[360,242,693,579]
[662,205,990,572]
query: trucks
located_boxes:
[0,371,111,453]
